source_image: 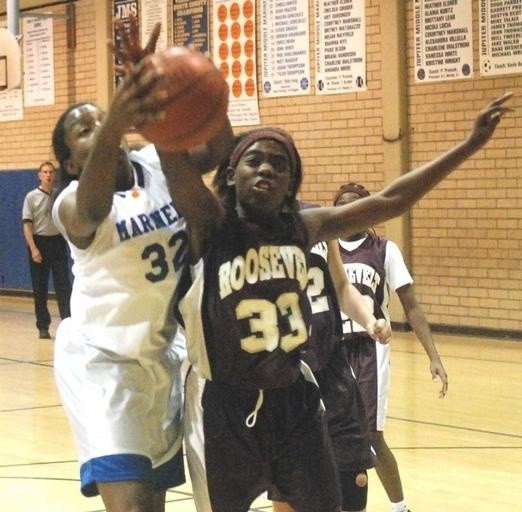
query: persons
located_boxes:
[105,10,511,511]
[52,56,234,512]
[22,162,72,338]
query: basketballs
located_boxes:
[134,47,228,152]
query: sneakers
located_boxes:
[39,327,49,338]
